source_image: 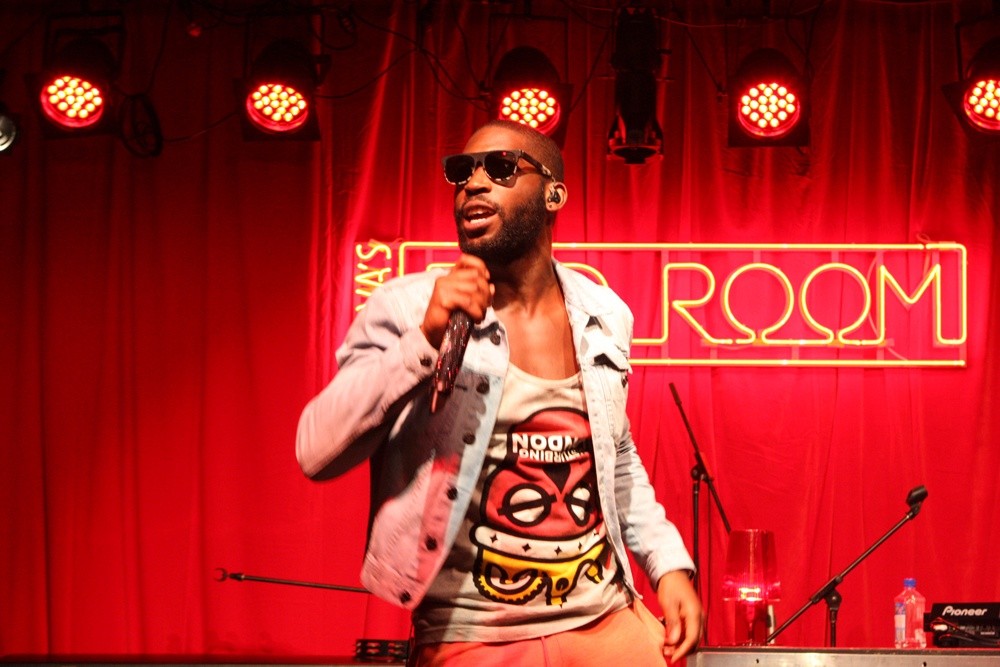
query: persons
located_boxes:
[295,117,705,666]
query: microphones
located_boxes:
[428,252,496,415]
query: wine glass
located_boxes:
[721,525,780,650]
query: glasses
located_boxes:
[442,149,557,183]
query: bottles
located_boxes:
[888,578,925,650]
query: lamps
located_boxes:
[0,2,1000,169]
